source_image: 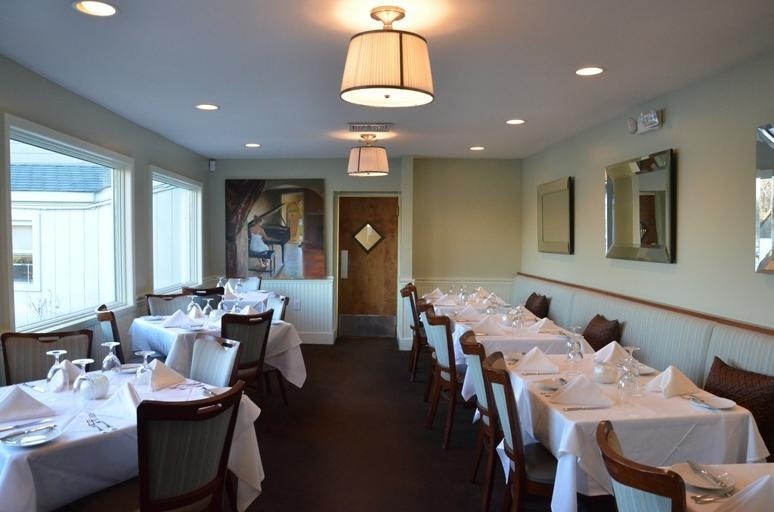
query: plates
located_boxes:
[7,425,61,447]
[203,385,245,398]
[669,461,740,493]
[689,394,736,410]
[146,315,167,322]
[536,377,568,389]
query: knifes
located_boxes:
[24,379,45,395]
[686,460,730,496]
[200,386,219,399]
[1,417,55,440]
[563,403,615,411]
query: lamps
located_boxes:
[625,117,638,134]
[345,131,391,177]
[339,5,435,108]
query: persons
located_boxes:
[250,214,273,280]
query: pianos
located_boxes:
[248,204,290,265]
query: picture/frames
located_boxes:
[536,175,574,255]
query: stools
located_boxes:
[247,249,276,278]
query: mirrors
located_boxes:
[352,219,385,255]
[755,124,774,274]
[603,148,677,263]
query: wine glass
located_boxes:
[44,341,156,406]
[564,324,642,400]
[186,274,259,328]
[445,283,550,338]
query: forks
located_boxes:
[86,411,116,436]
